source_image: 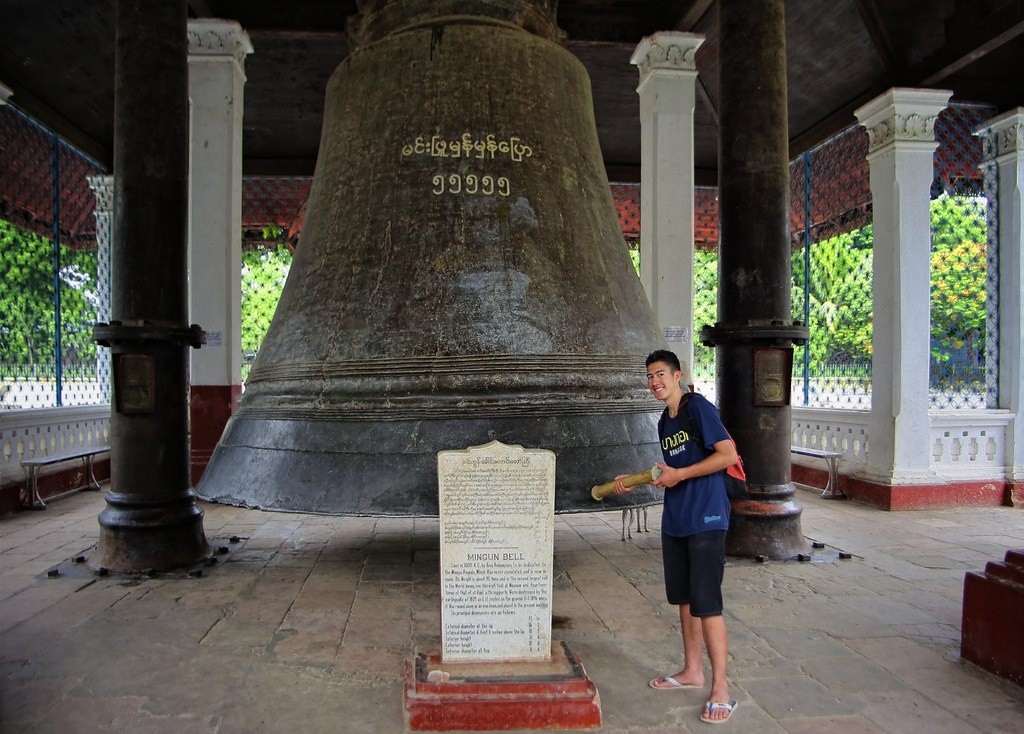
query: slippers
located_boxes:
[648,674,703,689]
[700,699,738,723]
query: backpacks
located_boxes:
[658,393,747,502]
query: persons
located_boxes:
[614,349,739,723]
